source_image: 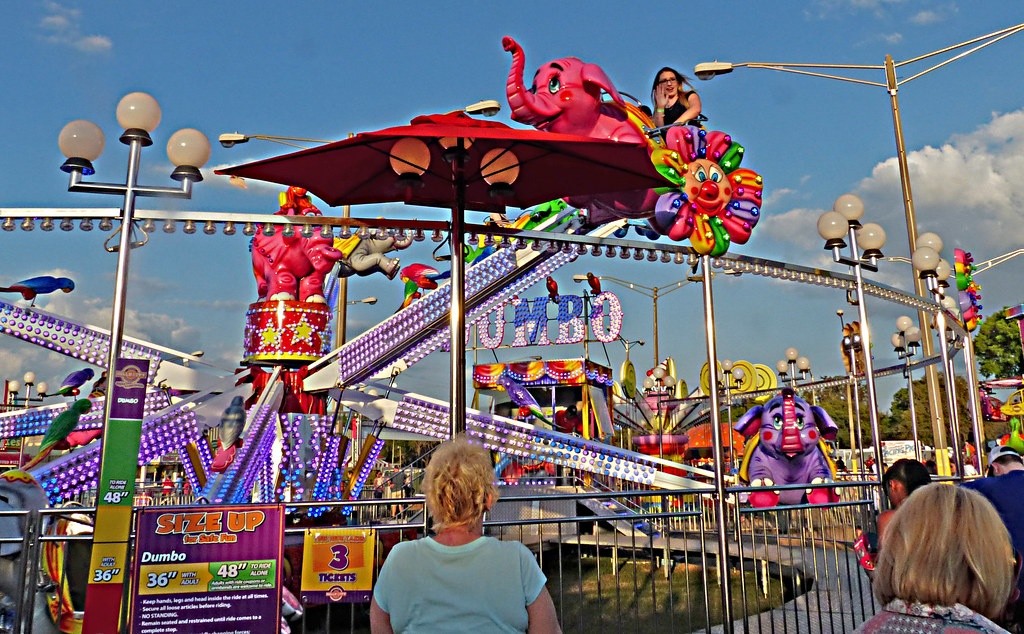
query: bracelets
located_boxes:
[656,106,667,114]
[654,114,666,118]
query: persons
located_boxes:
[555,401,592,435]
[490,212,510,227]
[370,436,562,634]
[514,406,532,422]
[835,439,1024,634]
[91,371,108,393]
[133,470,193,505]
[638,66,701,130]
[339,465,415,525]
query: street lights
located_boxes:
[9,370,50,470]
[779,349,810,394]
[572,268,748,429]
[818,194,888,513]
[217,96,500,357]
[694,21,1023,487]
[913,232,970,478]
[58,88,212,513]
[891,315,923,462]
[837,308,871,532]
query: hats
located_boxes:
[987,445,1022,477]
